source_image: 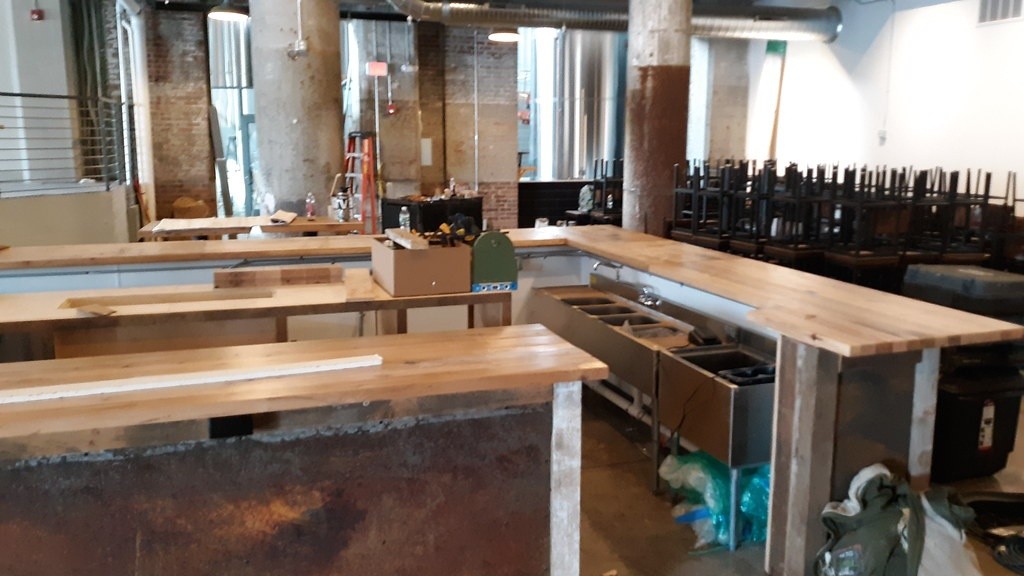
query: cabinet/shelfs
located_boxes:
[382,197,483,233]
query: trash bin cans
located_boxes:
[901,264,1024,483]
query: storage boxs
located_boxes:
[372,237,471,296]
[903,265,1024,367]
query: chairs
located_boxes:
[591,160,1024,284]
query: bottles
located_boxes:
[305,192,318,221]
[449,178,456,196]
[400,207,410,232]
[336,186,350,222]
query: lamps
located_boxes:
[488,27,520,42]
[208,0,248,21]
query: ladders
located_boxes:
[339,131,376,235]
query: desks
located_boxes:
[0,217,1024,576]
